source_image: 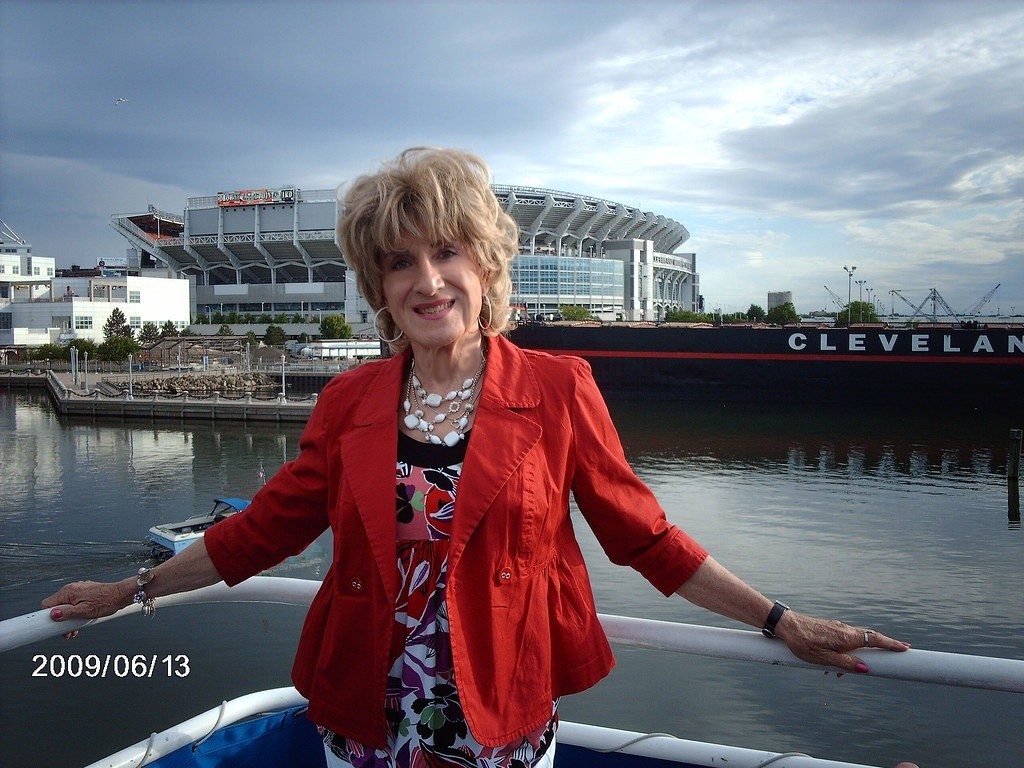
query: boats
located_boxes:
[143,496,254,559]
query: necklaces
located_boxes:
[404,346,489,447]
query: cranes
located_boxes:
[890,283,1001,322]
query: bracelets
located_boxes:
[133,567,157,620]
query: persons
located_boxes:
[41,145,912,768]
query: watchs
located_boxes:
[762,600,791,639]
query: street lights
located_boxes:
[865,288,873,323]
[843,266,857,326]
[127,352,133,396]
[280,353,286,405]
[69,345,81,386]
[874,293,886,318]
[83,349,89,394]
[855,280,866,323]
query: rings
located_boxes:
[864,630,875,648]
